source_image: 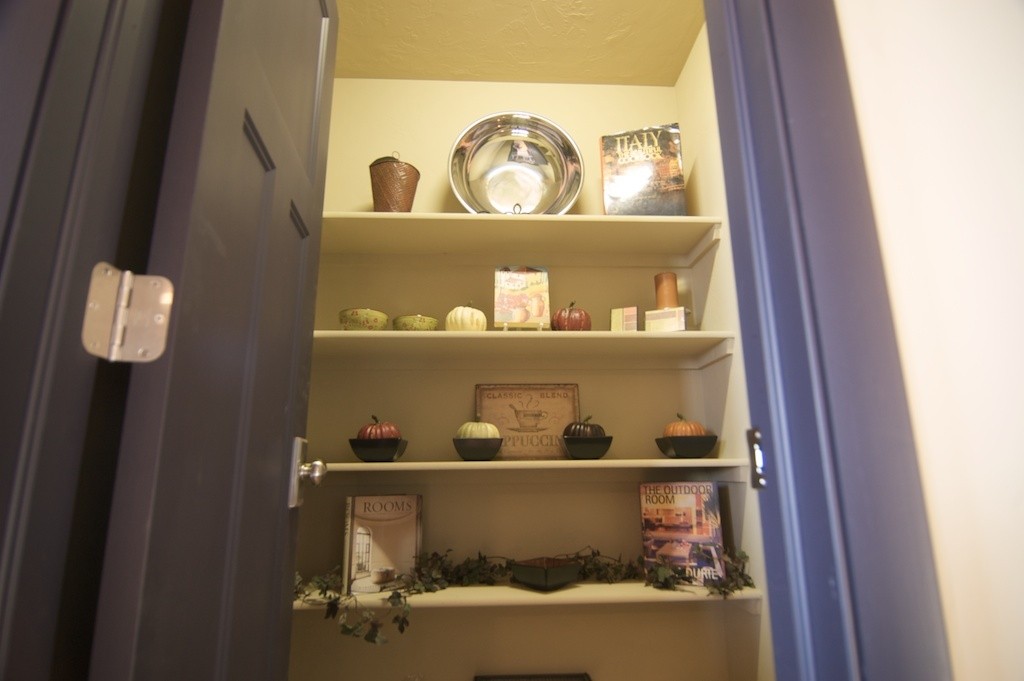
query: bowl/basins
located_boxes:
[349,439,408,462]
[393,315,440,331]
[511,557,579,591]
[448,111,584,214]
[453,438,503,461]
[655,435,717,458]
[560,435,612,460]
[339,308,389,330]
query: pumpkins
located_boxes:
[455,412,500,438]
[662,412,706,436]
[563,415,606,435]
[551,301,592,331]
[444,300,487,331]
[357,414,400,439]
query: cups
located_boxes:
[369,161,421,212]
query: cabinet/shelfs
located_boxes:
[295,213,760,607]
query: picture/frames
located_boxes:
[475,384,581,460]
